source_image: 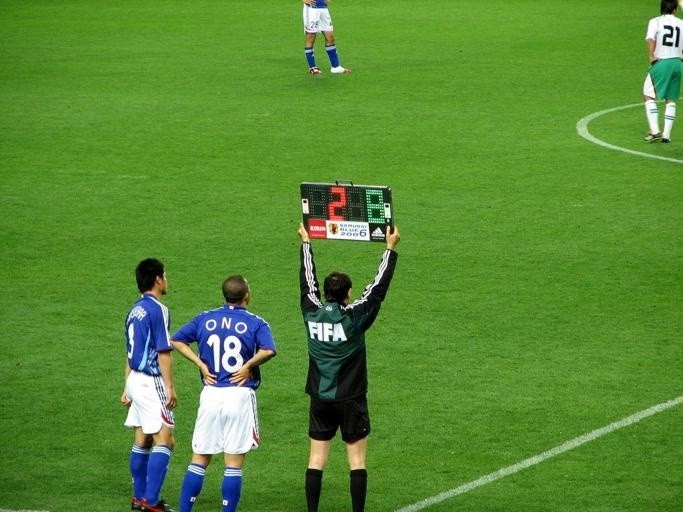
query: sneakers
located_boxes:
[331,65,351,74]
[644,131,671,143]
[309,67,322,75]
[131,496,174,512]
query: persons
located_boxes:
[642,0,683,143]
[302,1,352,76]
[297,221,400,512]
[169,275,277,512]
[120,259,177,512]
